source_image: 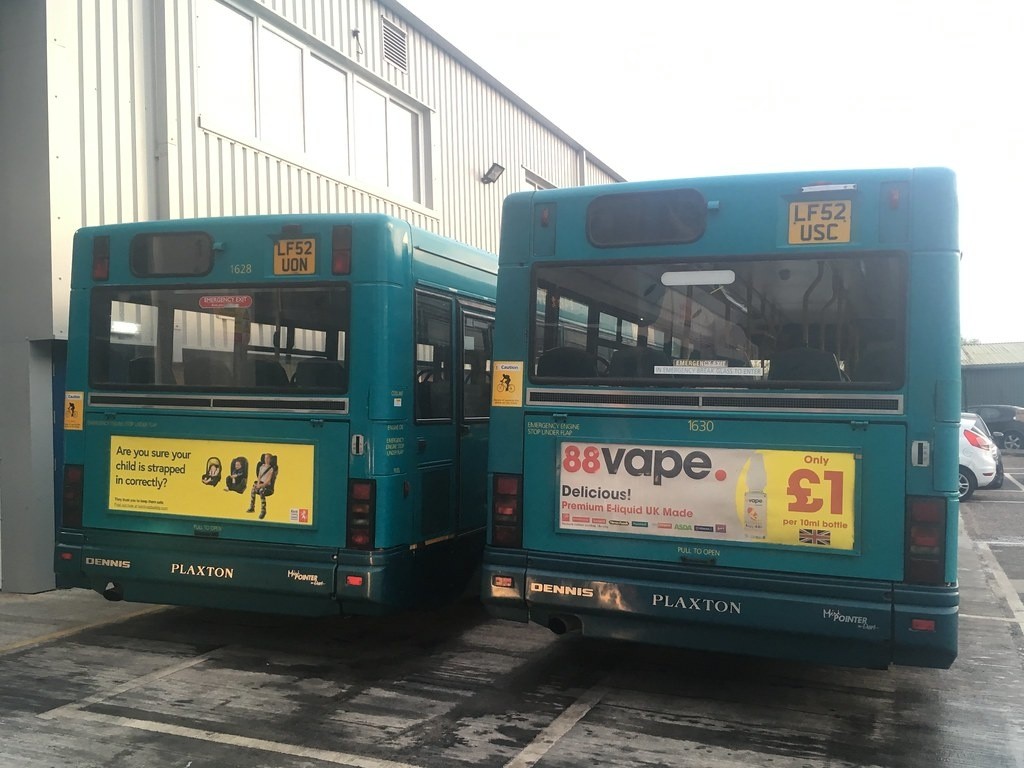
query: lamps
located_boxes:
[481,162,504,183]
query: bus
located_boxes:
[53,213,498,630]
[477,160,959,672]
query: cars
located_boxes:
[957,402,1024,505]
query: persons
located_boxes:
[202,466,220,483]
[224,460,243,493]
[247,453,273,519]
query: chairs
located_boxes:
[102,333,904,392]
